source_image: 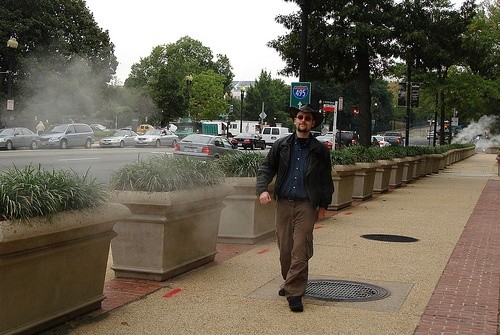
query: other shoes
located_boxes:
[287,295,304,312]
[278,284,288,296]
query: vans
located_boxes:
[136,125,154,135]
[259,127,293,147]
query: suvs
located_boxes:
[35,123,96,149]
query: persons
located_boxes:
[255,103,335,312]
[36,121,45,136]
[44,119,51,132]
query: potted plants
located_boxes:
[0,143,476,335]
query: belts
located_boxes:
[282,197,306,202]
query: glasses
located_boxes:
[297,114,311,121]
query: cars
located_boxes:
[99,129,140,148]
[174,133,239,163]
[231,133,266,150]
[426,125,461,145]
[134,129,179,148]
[90,124,107,131]
[0,127,40,150]
[310,130,405,150]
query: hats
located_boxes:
[288,104,324,129]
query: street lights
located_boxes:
[240,86,246,133]
[6,35,19,128]
[185,74,193,124]
